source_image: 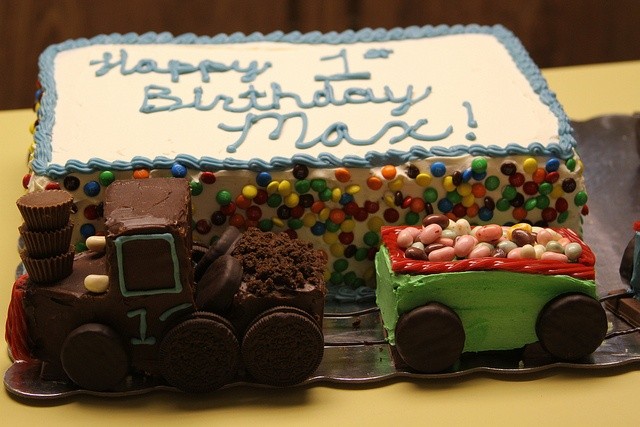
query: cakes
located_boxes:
[5,23,609,396]
